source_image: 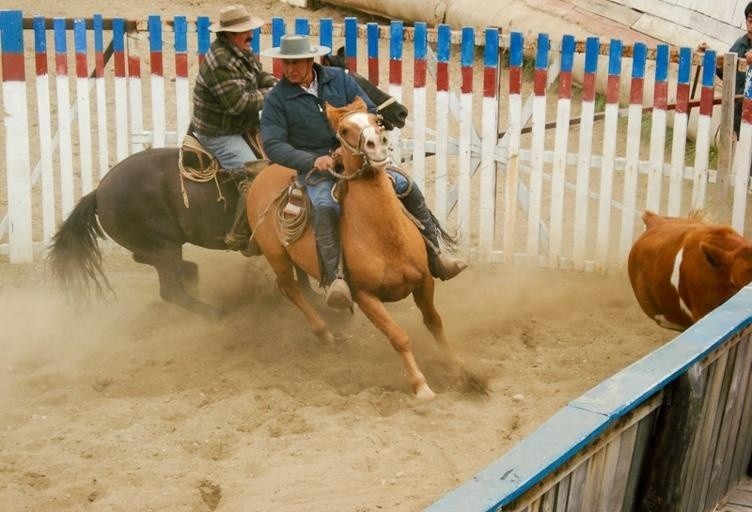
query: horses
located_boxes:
[46,46,410,322]
[245,94,461,401]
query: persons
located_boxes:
[259,35,468,310]
[189,4,281,248]
[696,1,752,141]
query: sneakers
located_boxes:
[225,232,249,249]
[430,253,468,281]
[325,276,352,310]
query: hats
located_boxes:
[207,4,265,33]
[259,35,331,60]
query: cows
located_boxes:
[627,212,751,331]
